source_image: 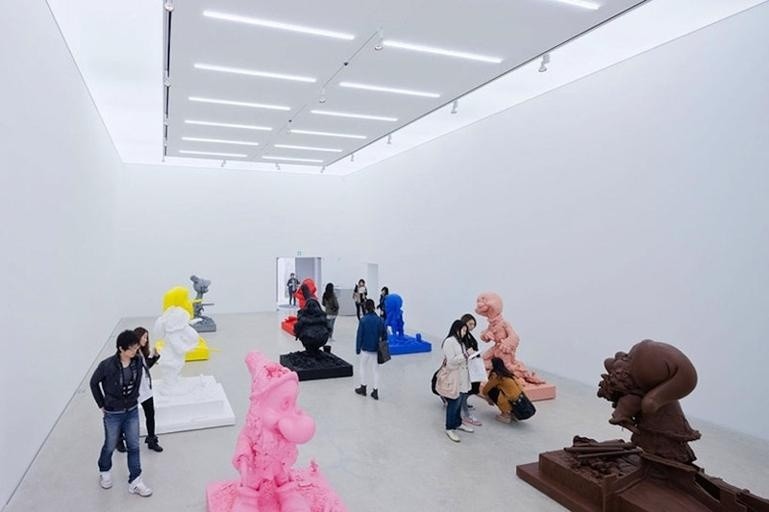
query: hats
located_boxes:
[243,350,299,400]
[631,338,698,415]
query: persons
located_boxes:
[91,330,152,496]
[377,287,388,320]
[435,320,474,442]
[322,282,339,341]
[355,299,388,400]
[115,327,163,452]
[287,273,301,308]
[353,279,368,321]
[432,314,482,426]
[483,357,536,425]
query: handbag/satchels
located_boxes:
[352,285,360,304]
[378,320,391,364]
[499,377,536,420]
[435,338,459,399]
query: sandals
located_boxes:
[496,414,511,423]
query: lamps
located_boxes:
[179,150,249,158]
[310,109,398,122]
[203,11,354,40]
[262,155,324,163]
[288,129,367,140]
[188,96,290,111]
[181,136,259,146]
[340,82,440,103]
[382,39,502,64]
[193,63,317,83]
[274,144,343,152]
[557,1,600,10]
[184,120,273,131]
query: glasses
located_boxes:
[127,344,140,350]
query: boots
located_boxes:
[355,385,366,395]
[116,437,126,452]
[146,436,163,452]
[371,389,378,400]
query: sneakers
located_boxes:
[463,416,481,426]
[446,429,459,442]
[457,423,473,432]
[100,470,112,488]
[129,477,151,497]
